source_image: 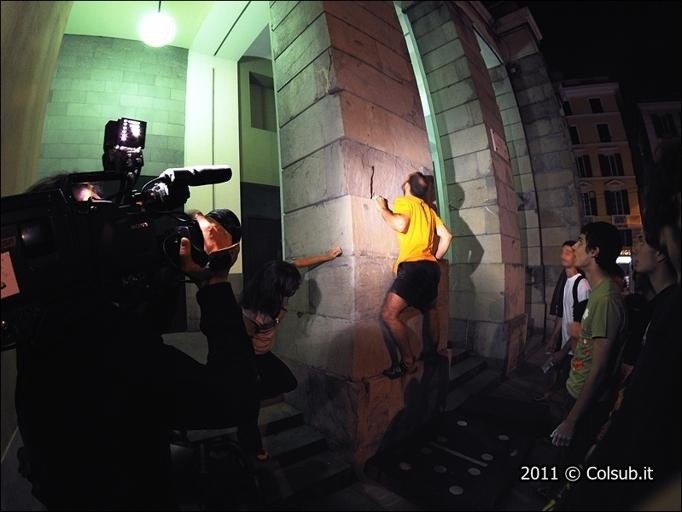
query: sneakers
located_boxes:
[383,357,420,379]
[256,447,273,463]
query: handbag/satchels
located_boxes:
[572,274,589,322]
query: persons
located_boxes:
[378,171,453,379]
[242,247,342,395]
[9,172,283,512]
[533,136,681,512]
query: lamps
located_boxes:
[133,0,182,49]
